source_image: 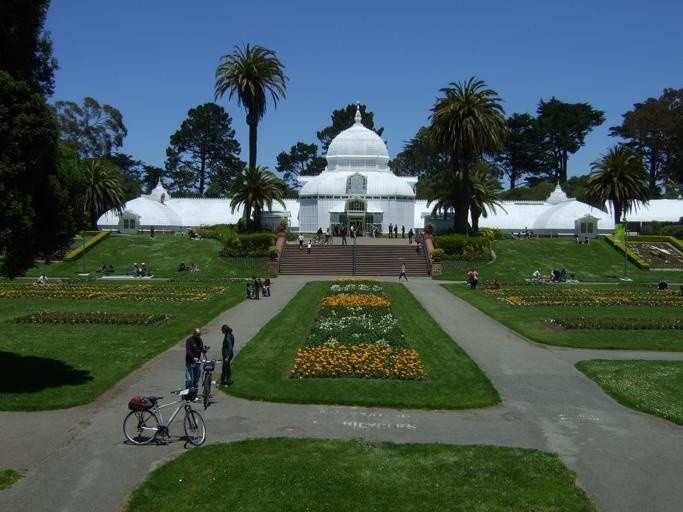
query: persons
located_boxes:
[141,261,146,277]
[297,221,419,255]
[261,277,271,297]
[183,327,208,405]
[188,261,197,271]
[575,236,581,249]
[583,237,589,247]
[215,323,236,388]
[35,271,50,284]
[175,260,187,272]
[531,263,567,286]
[467,266,478,290]
[149,225,156,240]
[132,261,138,278]
[252,274,260,299]
[245,283,251,299]
[398,262,408,283]
[509,226,537,241]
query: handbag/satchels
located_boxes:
[129,395,152,411]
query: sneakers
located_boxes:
[214,381,231,387]
[188,397,200,402]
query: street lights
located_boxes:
[622,217,627,280]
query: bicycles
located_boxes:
[193,358,223,410]
[123,383,207,446]
